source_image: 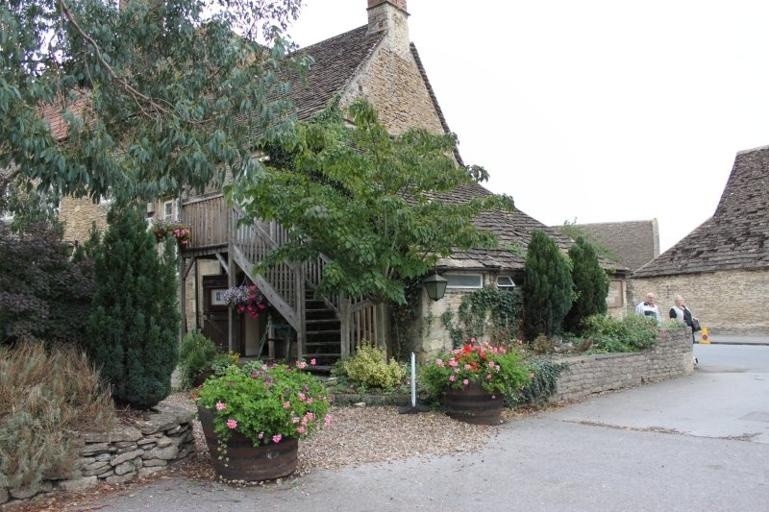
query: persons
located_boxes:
[636,293,659,317]
[670,295,695,343]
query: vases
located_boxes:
[439,385,504,427]
[198,405,300,483]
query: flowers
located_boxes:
[197,358,334,464]
[428,338,536,396]
[223,283,269,320]
[151,216,191,248]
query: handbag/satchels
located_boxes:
[692,318,700,331]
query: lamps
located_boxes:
[422,260,449,302]
